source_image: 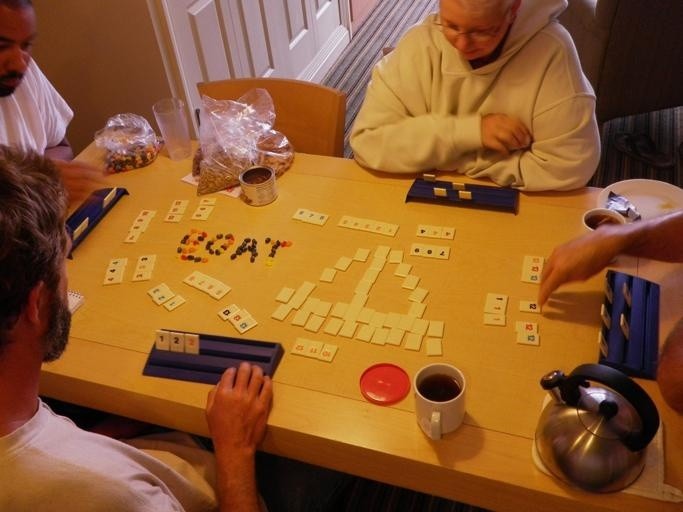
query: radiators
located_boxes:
[146,0,352,137]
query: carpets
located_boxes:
[327,0,438,158]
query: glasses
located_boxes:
[432,2,513,45]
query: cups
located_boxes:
[412,362,467,442]
[153,97,192,161]
[580,208,628,265]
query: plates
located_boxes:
[596,178,683,226]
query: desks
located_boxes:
[39,133,682,512]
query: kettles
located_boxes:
[533,361,661,494]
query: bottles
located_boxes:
[240,164,277,206]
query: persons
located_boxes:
[538,210,682,413]
[1,142,273,512]
[0,1,107,204]
[349,2,601,193]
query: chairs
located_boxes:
[195,78,347,157]
